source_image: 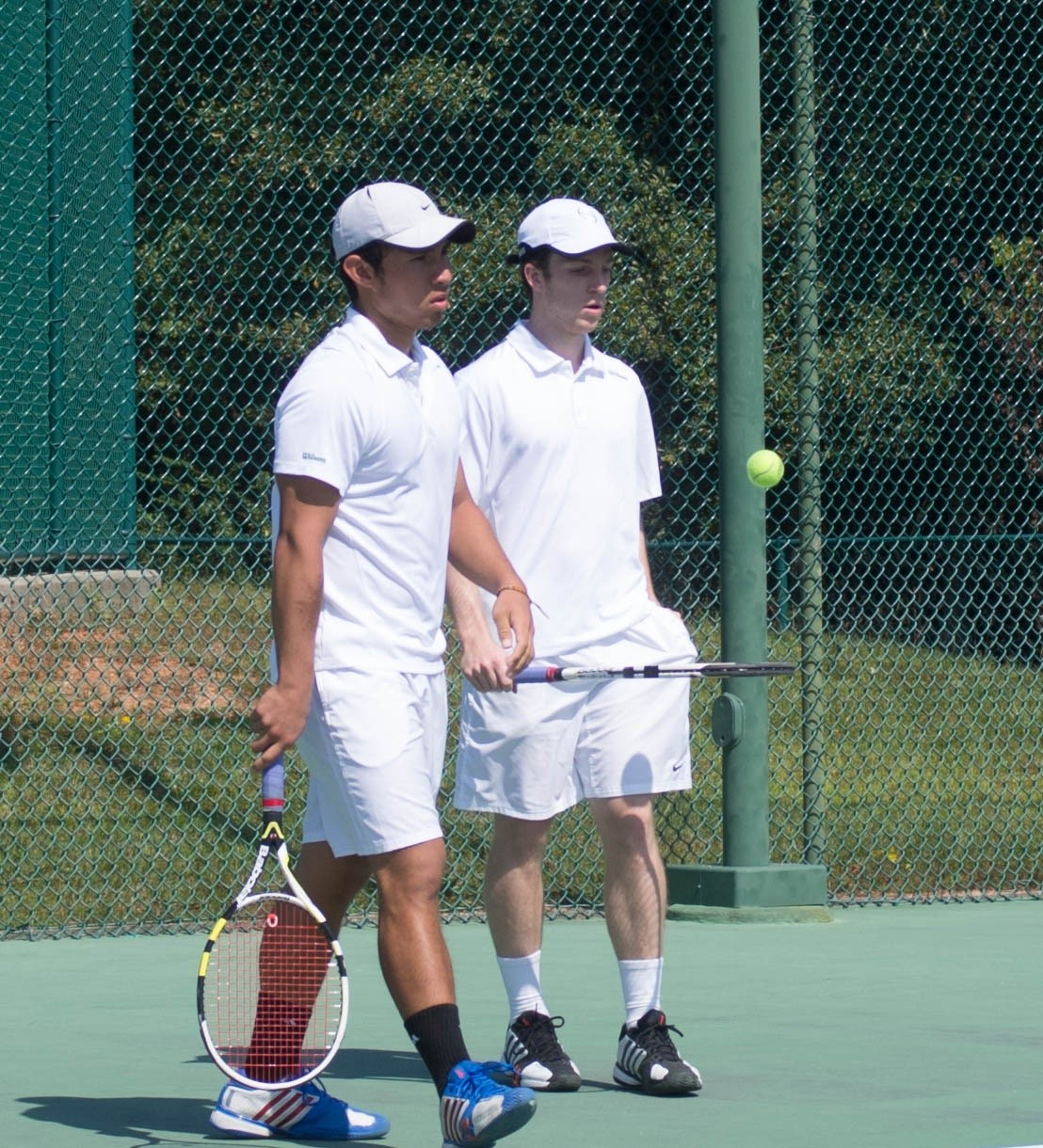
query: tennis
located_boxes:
[746,450,785,488]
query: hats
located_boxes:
[332,182,479,259]
[515,198,634,258]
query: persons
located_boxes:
[203,183,538,1146]
[447,200,709,1096]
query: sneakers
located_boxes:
[441,1061,537,1148]
[499,1003,584,1092]
[610,1010,703,1096]
[210,1074,393,1139]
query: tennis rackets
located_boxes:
[197,755,350,1092]
[511,662,797,684]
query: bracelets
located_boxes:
[497,585,549,619]
